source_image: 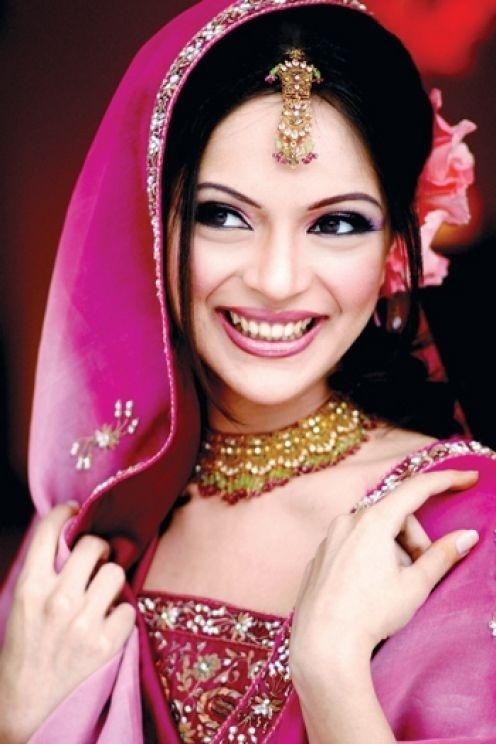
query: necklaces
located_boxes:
[184,389,390,507]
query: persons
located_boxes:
[0,0,496,744]
